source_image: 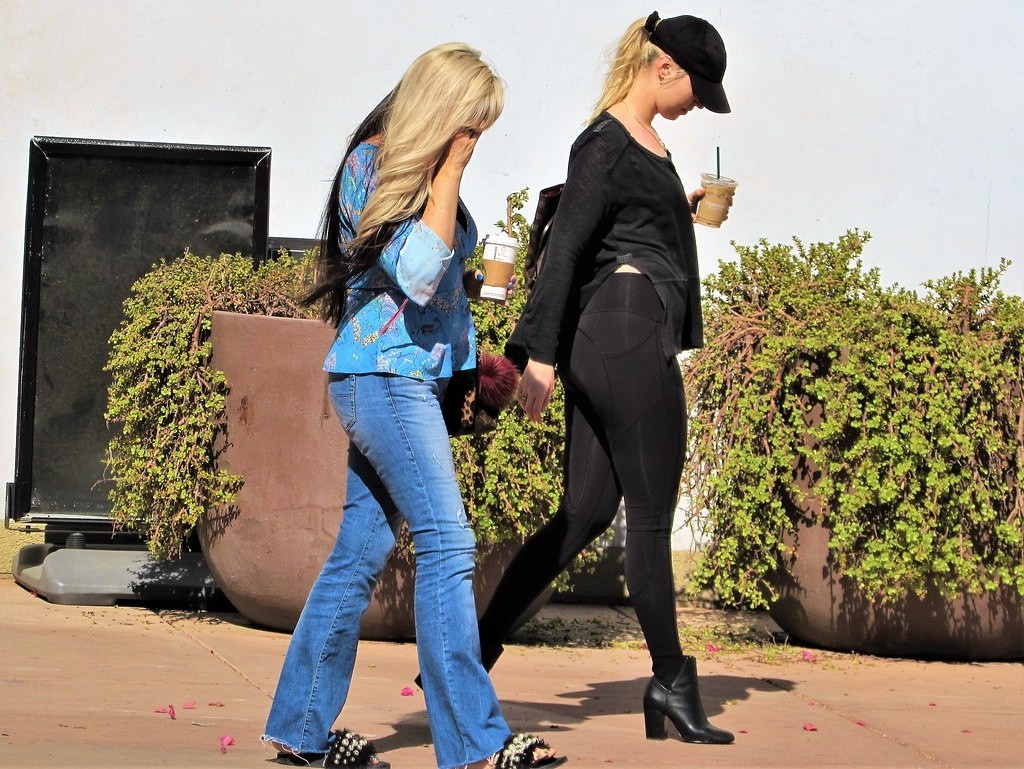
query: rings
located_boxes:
[519,393,526,401]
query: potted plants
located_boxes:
[97,188,1024,661]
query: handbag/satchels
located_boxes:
[522,182,566,298]
[440,349,520,435]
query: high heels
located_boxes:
[414,625,504,691]
[643,655,736,745]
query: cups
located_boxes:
[480,232,520,300]
[694,172,738,228]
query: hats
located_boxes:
[649,15,731,114]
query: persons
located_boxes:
[414,11,737,744]
[259,41,568,769]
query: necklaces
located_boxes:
[621,100,667,151]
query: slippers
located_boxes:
[493,732,568,769]
[276,728,391,769]
